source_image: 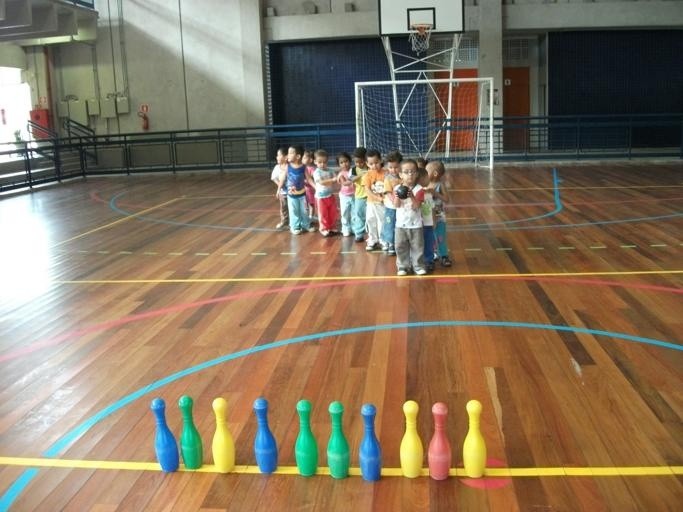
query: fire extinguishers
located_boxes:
[138,112,149,130]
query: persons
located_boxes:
[271,146,452,275]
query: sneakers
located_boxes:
[275,215,453,276]
